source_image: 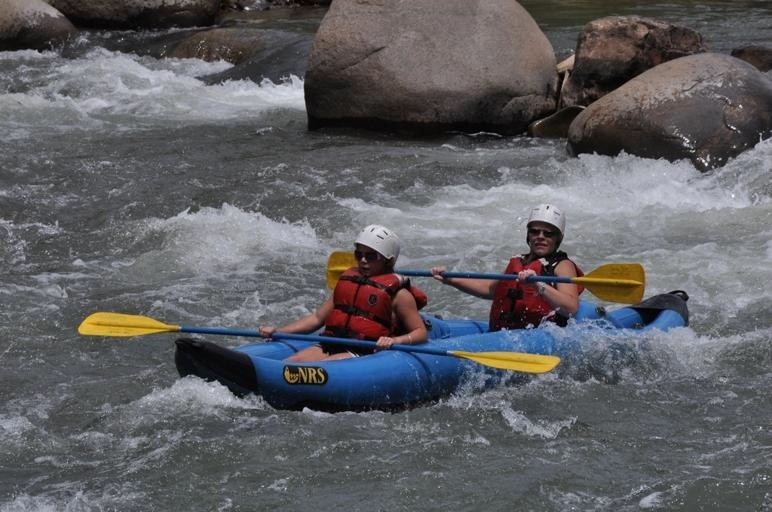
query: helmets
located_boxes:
[527,204,566,236]
[354,224,401,260]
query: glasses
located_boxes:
[529,228,556,238]
[354,249,380,262]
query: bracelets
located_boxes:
[406,332,413,345]
[534,282,546,297]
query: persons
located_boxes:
[259,223,428,363]
[430,205,586,328]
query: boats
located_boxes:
[172,288,689,416]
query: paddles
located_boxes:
[78,312,561,374]
[327,251,645,304]
